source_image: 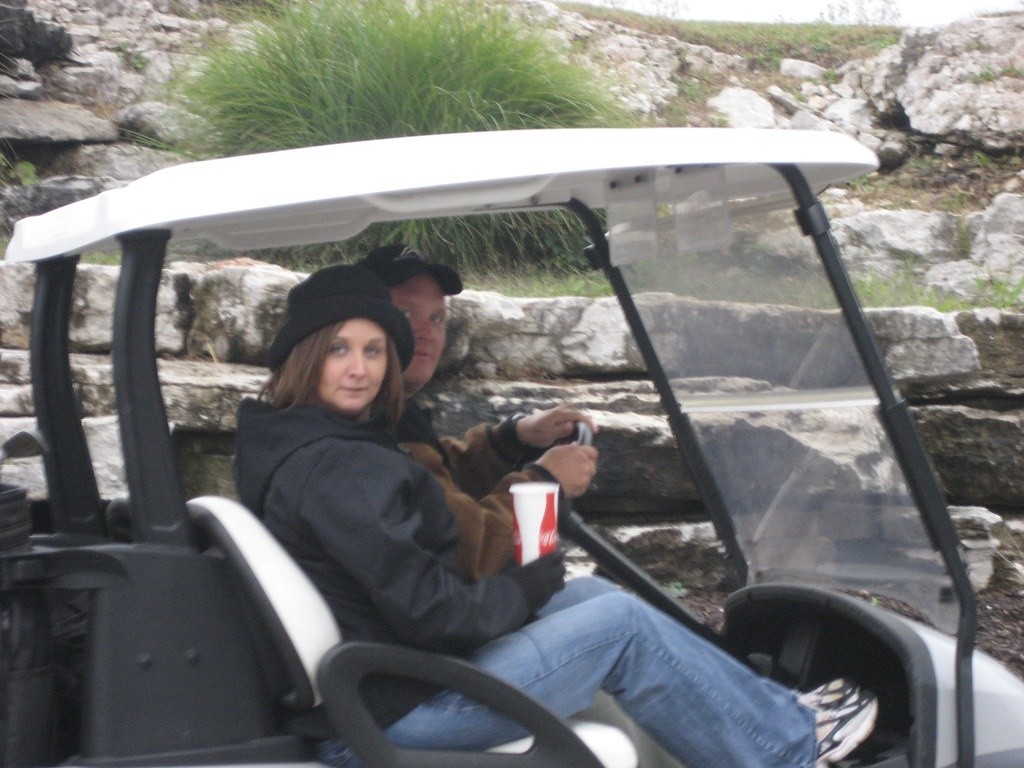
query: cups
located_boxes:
[509,483,559,566]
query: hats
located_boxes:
[268,265,414,374]
[353,245,462,296]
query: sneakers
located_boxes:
[813,690,879,768]
[796,679,852,714]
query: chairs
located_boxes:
[185,495,638,768]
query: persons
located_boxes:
[356,246,597,584]
[234,263,876,768]
